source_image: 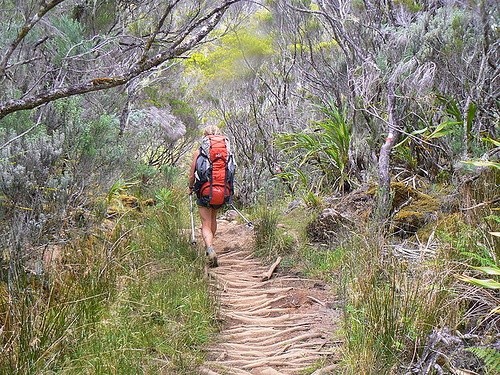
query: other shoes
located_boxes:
[207,249,219,267]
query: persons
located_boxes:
[188,124,235,268]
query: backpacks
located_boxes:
[192,133,237,209]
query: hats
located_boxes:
[203,125,222,138]
